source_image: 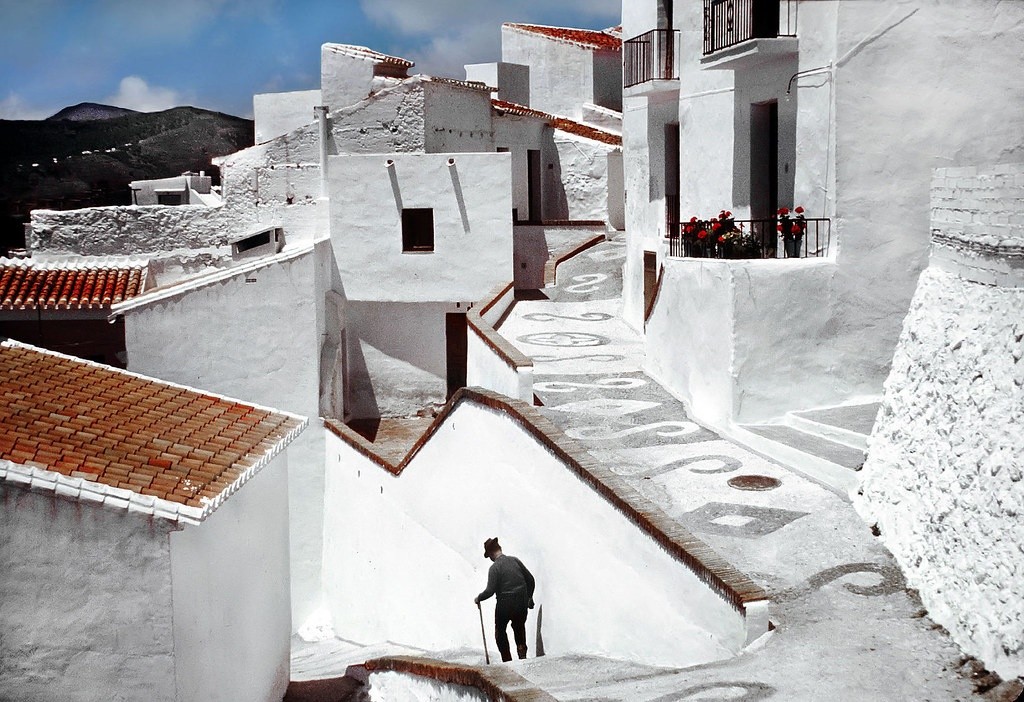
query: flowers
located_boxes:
[682,210,757,243]
[771,205,808,239]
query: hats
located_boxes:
[484,537,498,558]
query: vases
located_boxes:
[684,239,760,259]
[783,239,802,258]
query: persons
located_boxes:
[475,537,536,661]
